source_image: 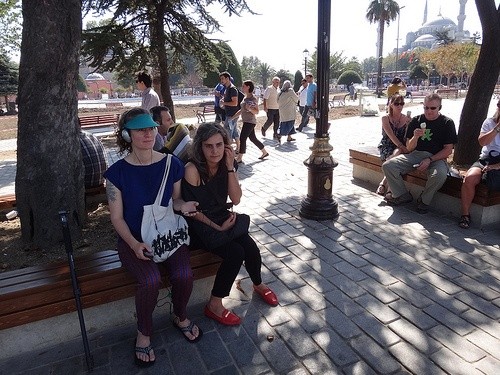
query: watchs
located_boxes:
[227,167,237,173]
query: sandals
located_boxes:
[459,214,472,228]
[375,183,393,202]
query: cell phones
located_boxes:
[183,209,208,214]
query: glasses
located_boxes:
[136,80,141,83]
[423,105,440,110]
[393,101,405,106]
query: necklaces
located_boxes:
[389,114,403,126]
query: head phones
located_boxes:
[118,107,158,143]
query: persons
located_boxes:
[180,121,280,329]
[136,70,161,114]
[77,116,111,193]
[261,76,283,141]
[218,70,240,154]
[296,74,318,133]
[102,108,202,365]
[385,77,407,112]
[295,78,310,127]
[143,104,175,157]
[376,93,410,199]
[346,81,357,101]
[234,81,269,162]
[380,94,455,213]
[458,96,500,229]
[277,80,299,142]
[213,75,235,129]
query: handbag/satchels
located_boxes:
[196,204,251,248]
[139,155,190,264]
[479,149,500,190]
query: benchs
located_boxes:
[195,104,216,122]
[326,94,348,107]
[349,142,499,230]
[78,113,121,130]
[1,241,221,330]
[435,88,458,99]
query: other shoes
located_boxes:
[252,282,280,305]
[203,305,241,327]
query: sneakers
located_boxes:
[275,134,281,142]
[236,159,242,164]
[391,192,413,206]
[296,127,302,132]
[261,128,266,136]
[273,133,279,137]
[416,201,430,214]
[258,152,269,160]
[287,138,296,141]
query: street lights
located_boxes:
[394,5,405,77]
[303,49,310,79]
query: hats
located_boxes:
[391,77,401,84]
[121,114,160,130]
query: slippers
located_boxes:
[172,314,203,341]
[132,332,157,368]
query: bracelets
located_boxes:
[428,156,434,164]
[396,142,402,148]
